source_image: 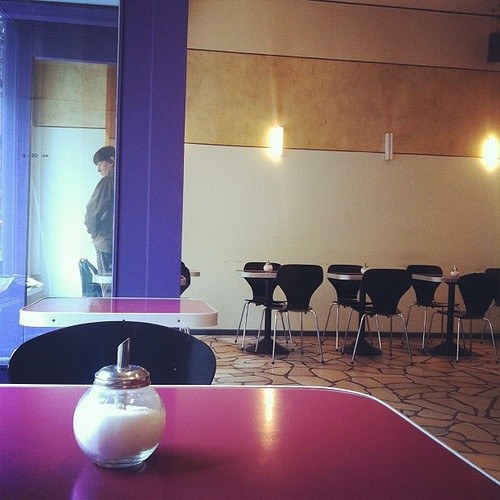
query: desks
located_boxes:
[326,272,382,356]
[236,270,290,354]
[19,297,218,329]
[0,384,500,500]
[412,274,471,356]
[91,272,200,297]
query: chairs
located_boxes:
[8,321,216,388]
[79,258,111,297]
[235,261,500,364]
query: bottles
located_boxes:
[264,260,273,273]
[361,263,369,274]
[72,337,167,469]
[450,265,460,278]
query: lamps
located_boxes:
[488,9,500,62]
[275,126,284,156]
[384,131,394,161]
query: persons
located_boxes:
[83,146,115,298]
